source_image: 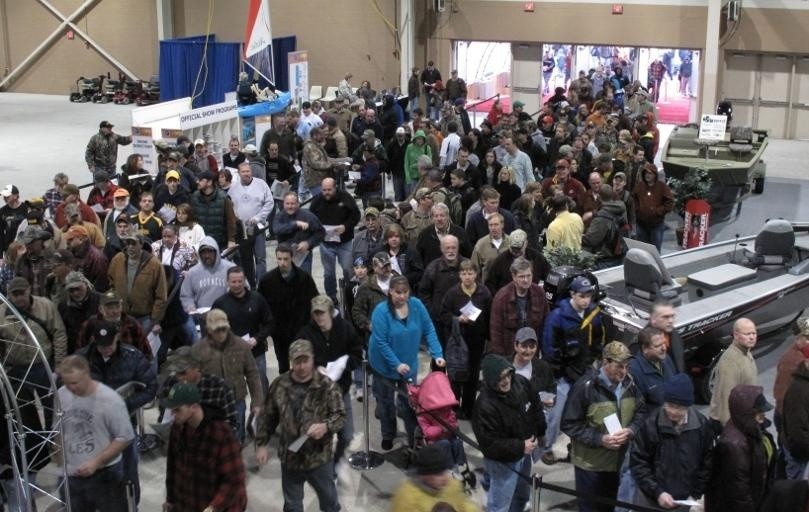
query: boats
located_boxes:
[536,216,808,407]
[659,117,772,196]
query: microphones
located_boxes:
[731,233,739,264]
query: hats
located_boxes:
[753,394,775,411]
[508,228,527,249]
[598,340,637,362]
[269,62,480,224]
[286,337,315,362]
[410,438,455,473]
[158,346,206,375]
[569,275,594,293]
[160,381,203,409]
[98,289,122,305]
[1,173,92,244]
[513,325,539,344]
[95,120,260,239]
[63,271,84,290]
[373,251,392,269]
[309,294,333,314]
[7,276,30,294]
[479,100,543,231]
[544,64,653,213]
[205,308,231,331]
[91,321,120,347]
[662,372,696,406]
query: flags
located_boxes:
[243,1,275,86]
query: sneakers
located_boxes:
[541,451,555,465]
[381,438,396,451]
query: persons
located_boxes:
[0,44,809,512]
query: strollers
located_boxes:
[398,363,477,490]
